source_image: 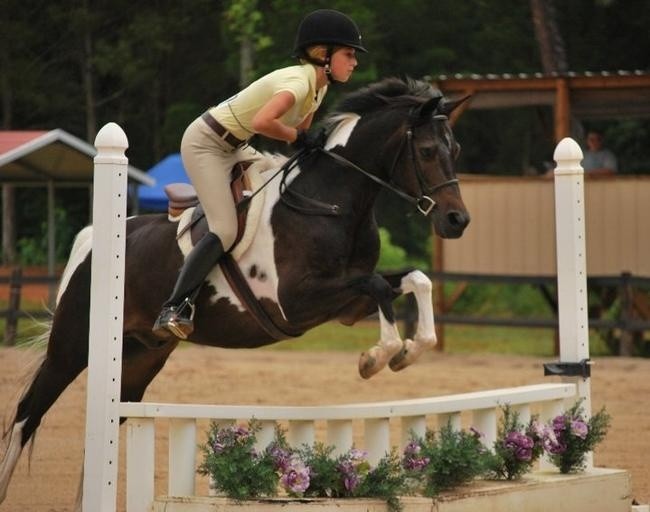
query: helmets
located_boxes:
[294,9,369,59]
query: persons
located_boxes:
[543,128,620,176]
[152,8,370,343]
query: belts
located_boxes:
[200,111,249,152]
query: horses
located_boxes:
[0,73,473,512]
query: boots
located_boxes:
[151,230,226,342]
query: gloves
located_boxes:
[288,126,330,149]
